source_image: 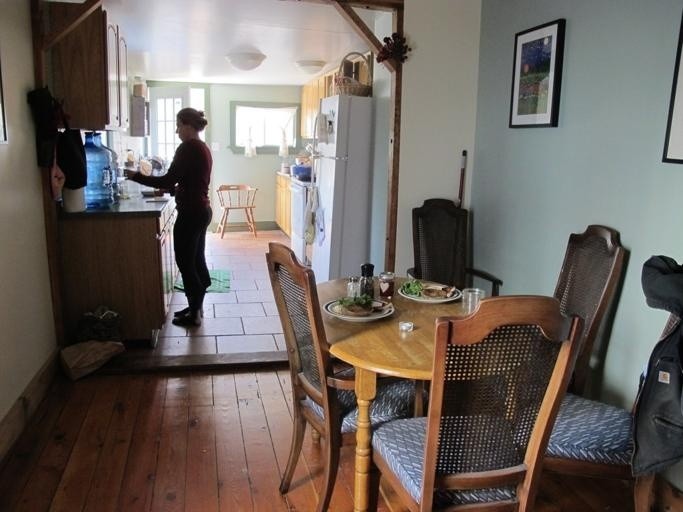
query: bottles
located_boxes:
[347,264,395,298]
[133,76,146,97]
[281,162,289,173]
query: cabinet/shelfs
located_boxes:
[130,97,151,136]
[301,75,334,139]
[56,198,176,346]
[31,0,128,131]
[276,172,291,238]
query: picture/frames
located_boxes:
[509,18,565,126]
[661,13,683,163]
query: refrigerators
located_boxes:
[311,96,372,283]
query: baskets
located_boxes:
[336,52,371,97]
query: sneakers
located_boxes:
[174,307,203,317]
[172,311,201,327]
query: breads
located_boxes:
[331,305,375,317]
[420,281,457,299]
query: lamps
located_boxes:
[225,53,266,71]
[299,59,327,74]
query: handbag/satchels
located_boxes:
[57,130,87,189]
[51,166,65,201]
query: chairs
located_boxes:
[554,226,630,396]
[408,199,503,297]
[266,242,428,512]
[372,295,584,511]
[216,185,259,239]
[543,256,683,512]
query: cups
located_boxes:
[461,288,485,315]
[117,166,128,200]
[399,331,414,340]
[400,321,414,331]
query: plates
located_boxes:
[323,299,394,322]
[397,283,462,303]
[141,191,154,196]
[146,196,168,202]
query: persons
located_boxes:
[124,108,213,327]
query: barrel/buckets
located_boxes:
[83,132,114,209]
[94,133,119,203]
